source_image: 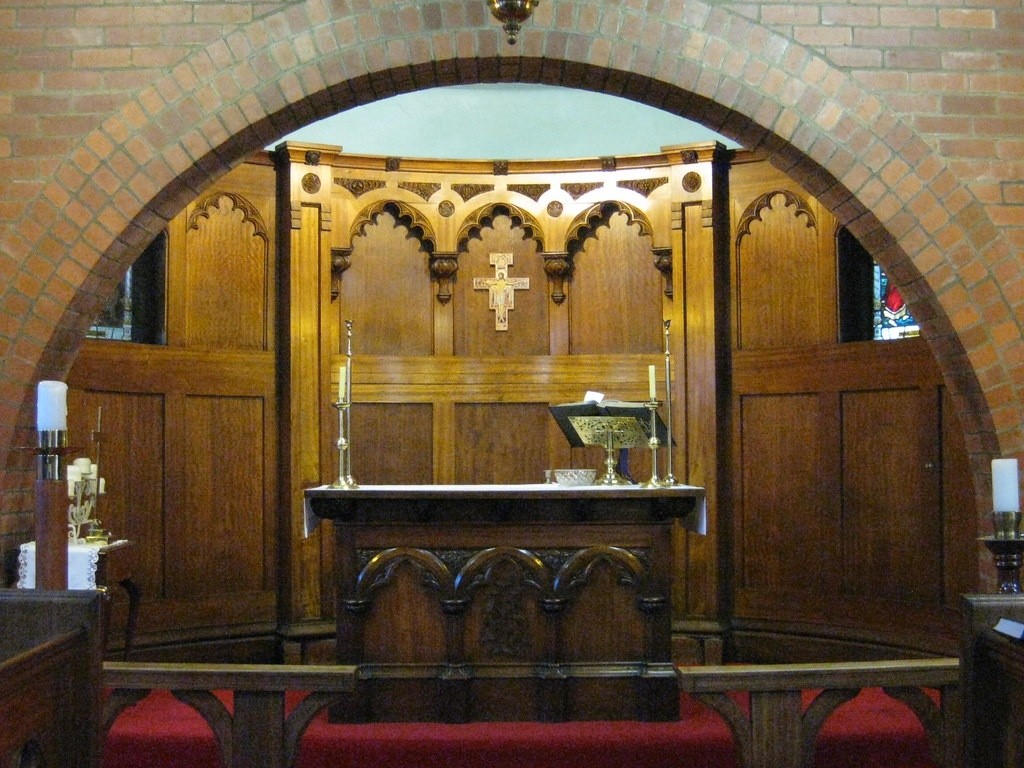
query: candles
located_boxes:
[991,458,1021,512]
[37,380,69,430]
[66,457,106,498]
[339,367,347,403]
[649,364,656,402]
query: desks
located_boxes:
[303,481,708,723]
[17,537,140,663]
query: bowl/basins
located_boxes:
[544,470,597,486]
